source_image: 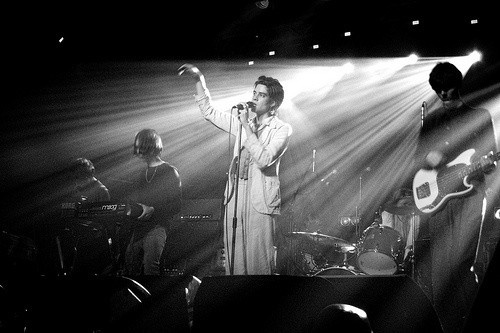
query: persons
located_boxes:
[413,62,497,314]
[381,189,419,259]
[177,63,293,276]
[53,158,110,202]
[122,129,181,275]
[313,303,373,333]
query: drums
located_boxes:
[356,224,405,276]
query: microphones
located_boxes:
[312,149,317,173]
[358,167,371,178]
[320,170,338,183]
[233,101,254,109]
[421,104,425,127]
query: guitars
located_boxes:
[413,147,500,216]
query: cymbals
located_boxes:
[286,231,357,254]
[382,196,418,215]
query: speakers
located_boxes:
[192,274,447,333]
[159,198,227,276]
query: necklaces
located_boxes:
[146,163,158,183]
